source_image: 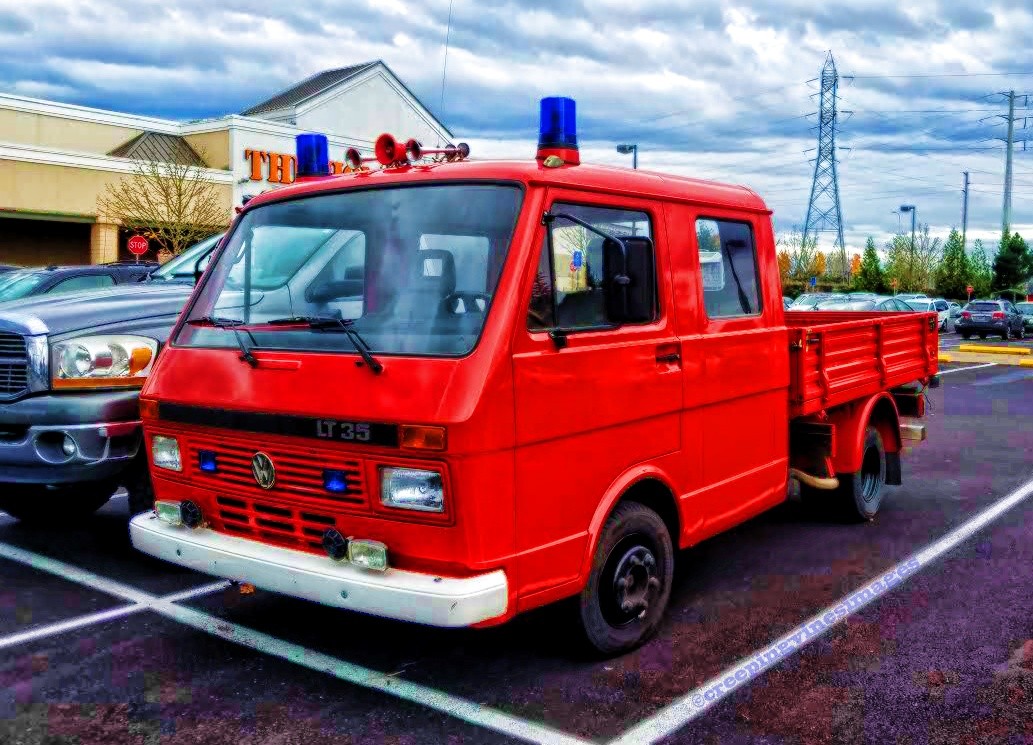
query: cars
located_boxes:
[782,291,962,335]
[1014,302,1033,331]
[954,299,1026,341]
[0,258,164,312]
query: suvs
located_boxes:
[0,218,491,533]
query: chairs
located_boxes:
[554,236,610,326]
[386,249,456,321]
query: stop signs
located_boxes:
[128,236,148,255]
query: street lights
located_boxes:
[900,204,916,291]
[617,143,638,237]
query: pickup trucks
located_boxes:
[127,93,943,659]
